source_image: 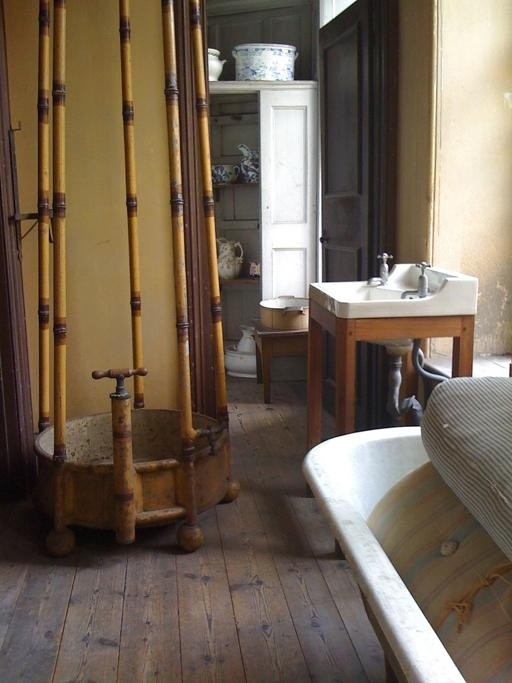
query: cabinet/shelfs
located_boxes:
[206,78,322,299]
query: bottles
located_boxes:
[207,47,228,81]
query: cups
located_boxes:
[211,164,240,186]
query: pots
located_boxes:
[232,44,299,83]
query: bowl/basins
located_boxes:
[258,293,312,332]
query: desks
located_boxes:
[250,320,328,403]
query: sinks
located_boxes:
[309,262,478,320]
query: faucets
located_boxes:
[366,253,394,285]
[401,262,432,298]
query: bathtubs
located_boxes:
[300,421,512,682]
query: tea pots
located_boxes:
[236,144,260,184]
